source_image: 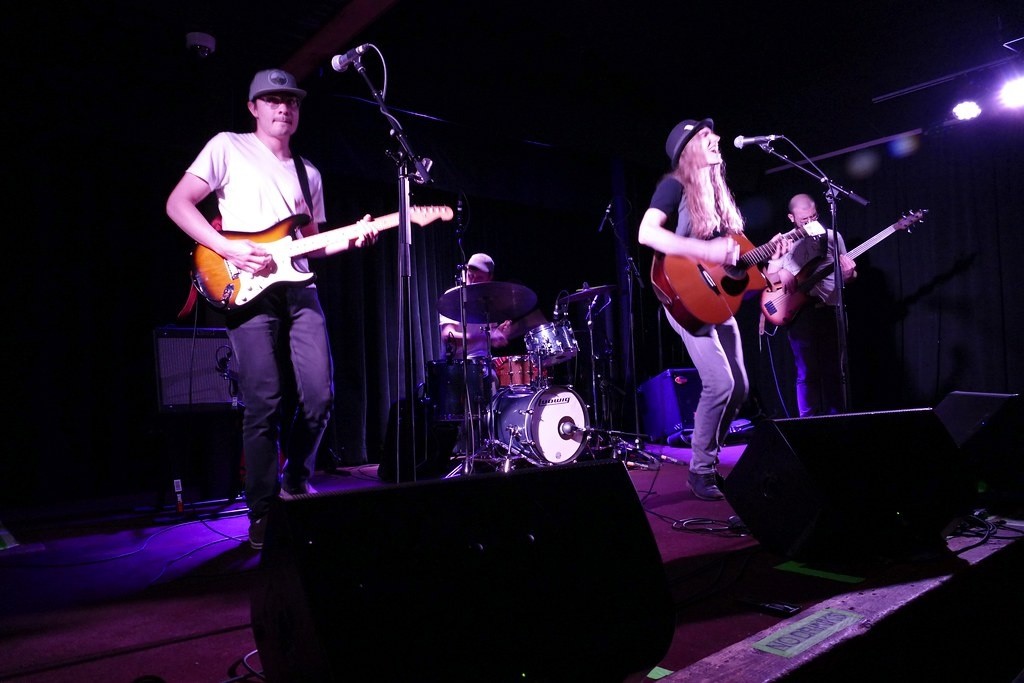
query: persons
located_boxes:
[758,192,857,416]
[638,118,748,498]
[439,253,513,391]
[167,68,378,551]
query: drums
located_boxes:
[492,354,548,389]
[425,358,487,423]
[523,320,580,367]
[486,384,591,465]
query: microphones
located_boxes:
[331,43,370,72]
[598,204,612,231]
[585,295,599,320]
[215,351,232,373]
[553,300,560,320]
[458,195,463,212]
[559,421,578,436]
[733,135,784,149]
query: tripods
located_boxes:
[443,212,686,478]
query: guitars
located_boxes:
[759,207,930,328]
[649,220,828,338]
[187,203,455,320]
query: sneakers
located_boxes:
[278,482,317,498]
[687,468,724,501]
[248,518,267,546]
[714,471,726,490]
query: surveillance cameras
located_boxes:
[185,33,216,58]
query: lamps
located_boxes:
[951,76,982,121]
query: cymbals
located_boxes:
[560,284,618,304]
[438,281,539,325]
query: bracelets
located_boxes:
[704,239,710,263]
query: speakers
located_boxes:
[636,368,702,439]
[724,390,1024,563]
[152,327,244,416]
[246,461,679,683]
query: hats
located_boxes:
[249,70,307,102]
[666,117,713,166]
[468,252,495,276]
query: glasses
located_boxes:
[258,96,301,111]
[793,213,819,225]
